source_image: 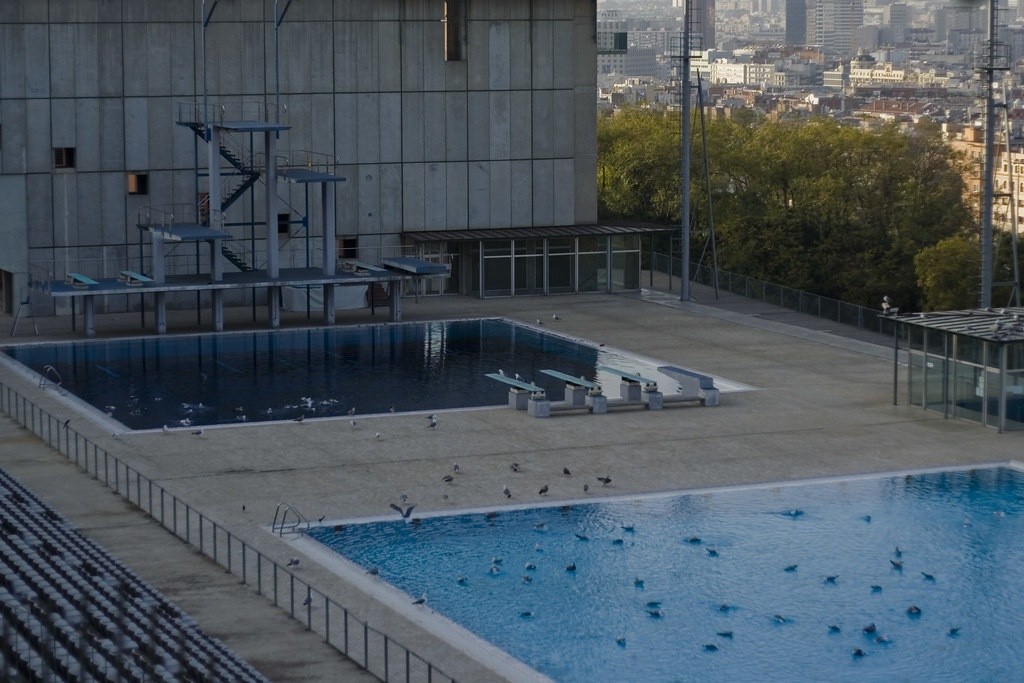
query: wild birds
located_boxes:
[387,462,612,519]
[702,604,791,650]
[821,573,840,583]
[827,622,889,657]
[497,368,526,382]
[454,518,665,648]
[535,311,562,325]
[907,601,962,637]
[685,534,721,556]
[881,292,1024,336]
[781,564,799,574]
[104,388,438,441]
[868,545,935,592]
[783,508,874,522]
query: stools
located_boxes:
[0,468,272,683]
